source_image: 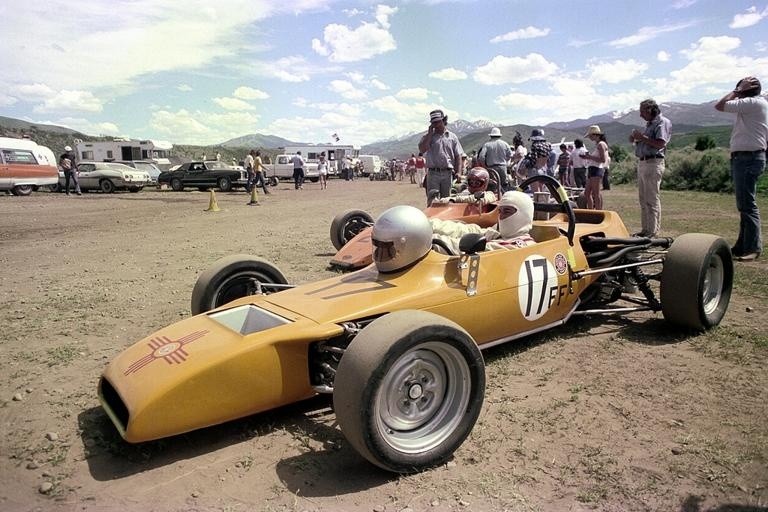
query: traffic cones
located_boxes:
[247,182,260,206]
[202,188,223,212]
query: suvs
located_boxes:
[0,137,60,196]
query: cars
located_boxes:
[48,160,269,193]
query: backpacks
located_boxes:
[410,159,414,165]
[62,158,71,169]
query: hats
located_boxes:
[529,129,546,141]
[583,125,605,138]
[429,110,444,122]
[488,127,502,137]
[64,146,72,151]
[462,153,467,157]
[419,153,423,156]
[511,146,514,149]
[393,157,396,160]
[513,136,522,145]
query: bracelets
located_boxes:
[733,85,745,96]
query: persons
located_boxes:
[318,156,328,190]
[628,98,673,237]
[479,127,513,192]
[289,151,305,189]
[250,151,272,194]
[579,124,609,210]
[428,190,537,255]
[245,149,255,195]
[232,157,237,165]
[440,166,497,206]
[713,75,768,262]
[524,129,551,200]
[417,110,464,207]
[341,131,612,192]
[214,151,221,160]
[60,146,82,196]
[200,151,207,161]
[238,157,244,166]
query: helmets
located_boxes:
[371,205,433,275]
[467,166,490,193]
[498,191,534,239]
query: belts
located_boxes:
[640,154,664,161]
[429,167,452,171]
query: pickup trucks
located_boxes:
[257,154,322,182]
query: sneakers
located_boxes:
[731,247,761,260]
[633,230,658,239]
